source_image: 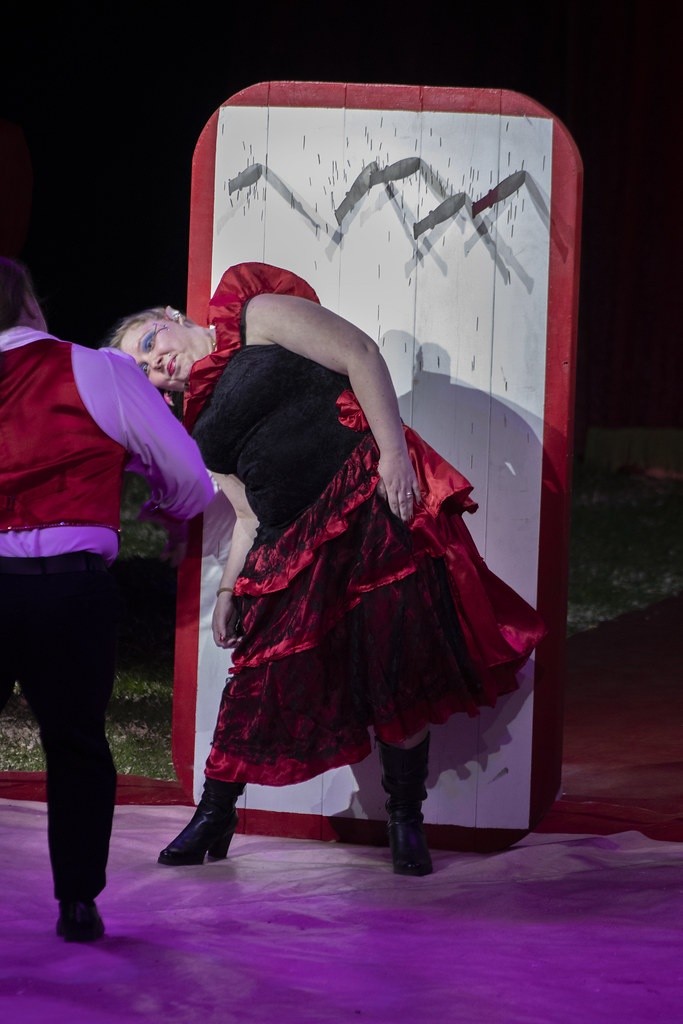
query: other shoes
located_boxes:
[55,896,106,944]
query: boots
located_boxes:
[372,731,433,878]
[158,778,247,865]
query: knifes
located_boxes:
[334,162,380,227]
[411,193,466,240]
[228,164,263,196]
[471,169,526,220]
[368,157,421,189]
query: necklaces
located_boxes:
[207,333,215,353]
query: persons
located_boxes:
[109,261,549,878]
[0,255,217,940]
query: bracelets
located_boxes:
[216,587,232,596]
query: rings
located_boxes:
[406,492,412,496]
